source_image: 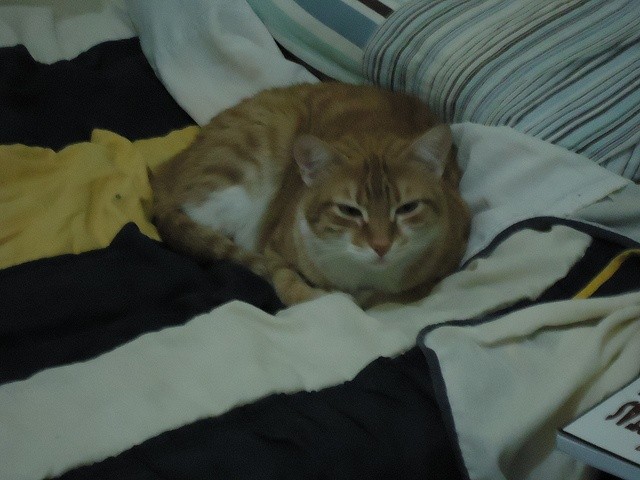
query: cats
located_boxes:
[142,81,473,311]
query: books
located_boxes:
[554,370,640,479]
